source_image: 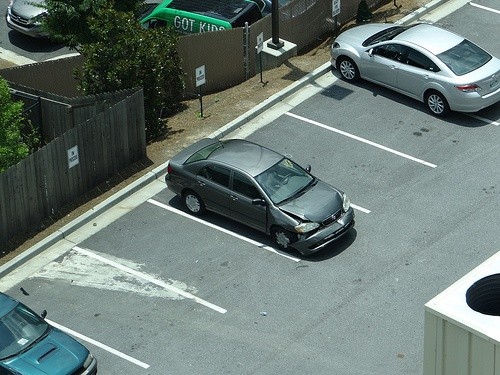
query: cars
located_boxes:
[6,0,53,38]
[166,138,355,256]
[259,0,325,31]
[0,292,98,375]
[329,21,500,117]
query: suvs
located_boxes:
[131,1,266,38]
